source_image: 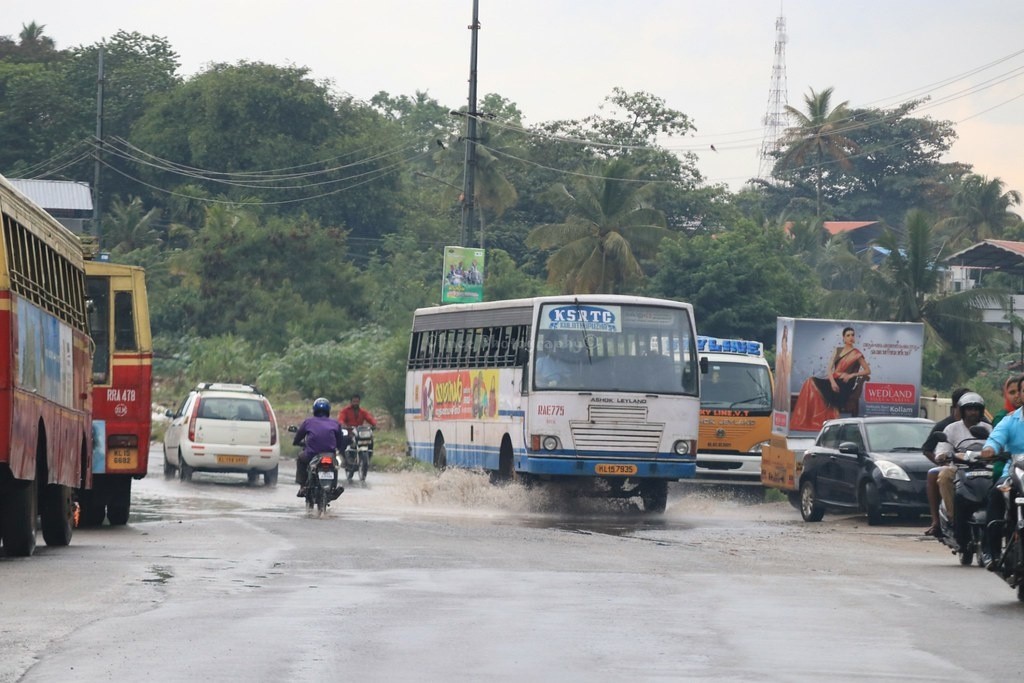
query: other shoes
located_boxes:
[296,489,307,497]
[924,522,939,536]
[981,551,1000,570]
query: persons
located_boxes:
[990,376,1022,484]
[980,373,1024,564]
[292,398,344,497]
[337,395,377,468]
[790,327,871,430]
[446,257,483,285]
[921,387,989,535]
[934,392,993,528]
[773,325,790,412]
[536,340,569,386]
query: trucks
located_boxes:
[83,259,153,528]
[0,172,97,557]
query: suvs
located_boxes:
[163,382,281,485]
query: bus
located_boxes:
[632,336,775,502]
[404,294,709,515]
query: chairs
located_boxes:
[234,405,254,420]
[203,407,218,418]
[417,346,527,365]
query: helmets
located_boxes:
[312,398,331,418]
[957,392,985,409]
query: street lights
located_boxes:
[414,170,486,250]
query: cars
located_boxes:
[798,417,938,525]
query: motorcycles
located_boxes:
[760,406,928,509]
[924,431,1006,565]
[342,424,376,480]
[288,425,349,515]
[968,425,1024,602]
